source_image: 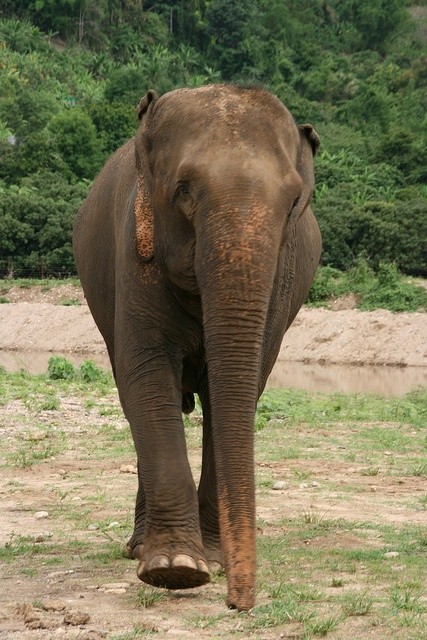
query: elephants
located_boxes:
[70,79,325,615]
